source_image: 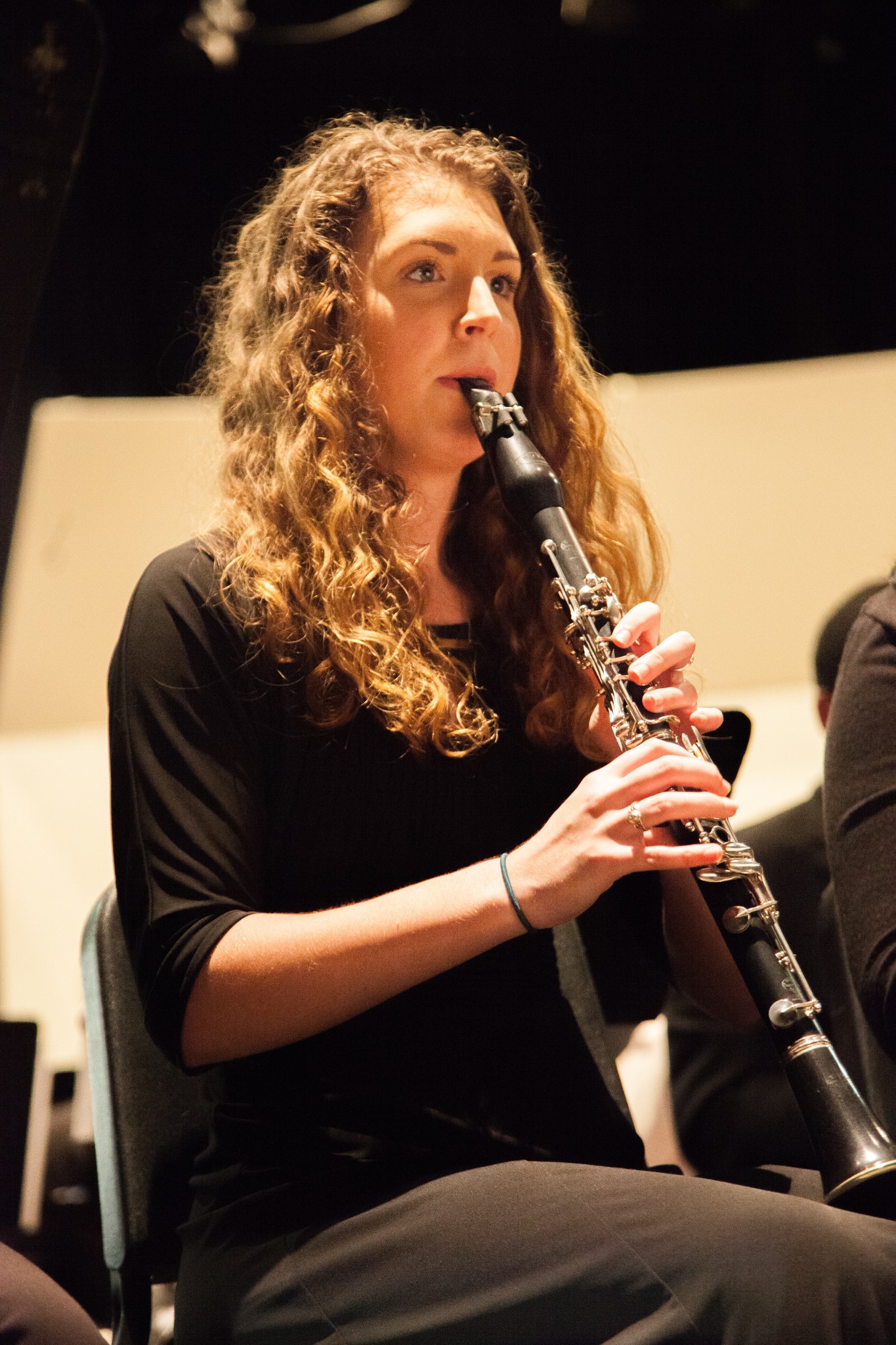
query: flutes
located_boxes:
[456,368,895,1218]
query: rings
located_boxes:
[627,802,652,832]
[668,653,693,673]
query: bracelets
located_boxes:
[497,850,538,934]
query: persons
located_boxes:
[820,589,894,1159]
[95,112,896,1345]
[669,581,888,1205]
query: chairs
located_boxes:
[80,879,637,1345]
